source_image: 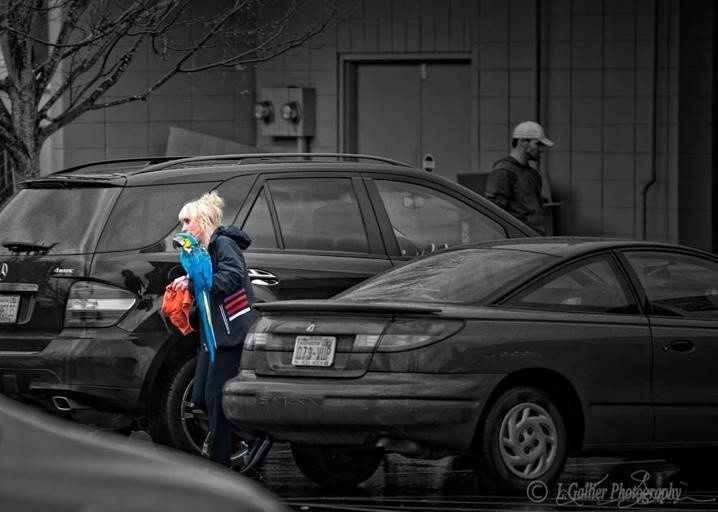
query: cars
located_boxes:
[0,153,717,511]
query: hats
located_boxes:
[512,121,555,148]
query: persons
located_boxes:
[487,121,555,238]
[171,190,257,468]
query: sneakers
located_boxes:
[239,439,270,473]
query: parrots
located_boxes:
[171,232,219,363]
